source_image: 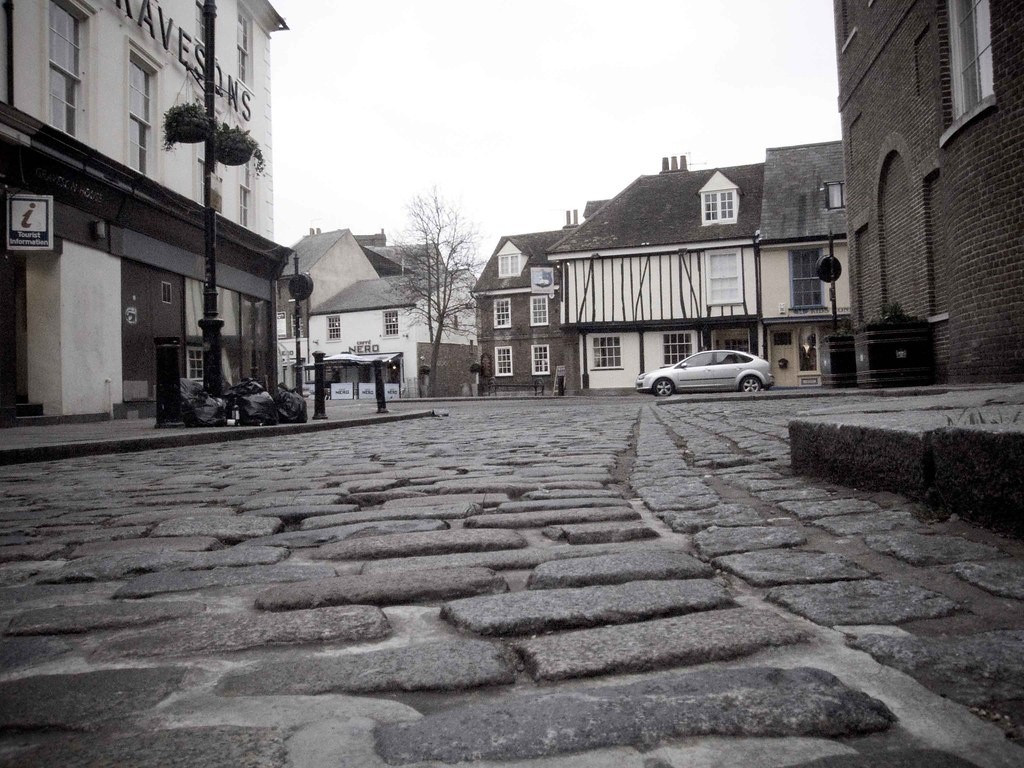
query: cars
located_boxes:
[634,349,774,398]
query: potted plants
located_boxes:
[818,318,857,389]
[853,300,935,388]
[214,123,267,178]
[161,97,213,152]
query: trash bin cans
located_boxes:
[154,336,186,428]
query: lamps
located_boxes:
[94,219,105,240]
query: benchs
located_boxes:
[488,377,545,397]
